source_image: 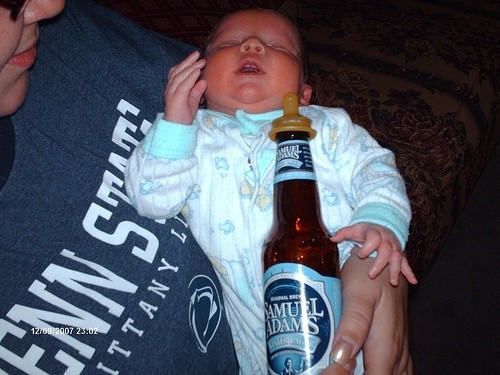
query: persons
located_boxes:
[125,6,417,375]
[0,0,414,375]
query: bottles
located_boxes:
[261,92,341,375]
